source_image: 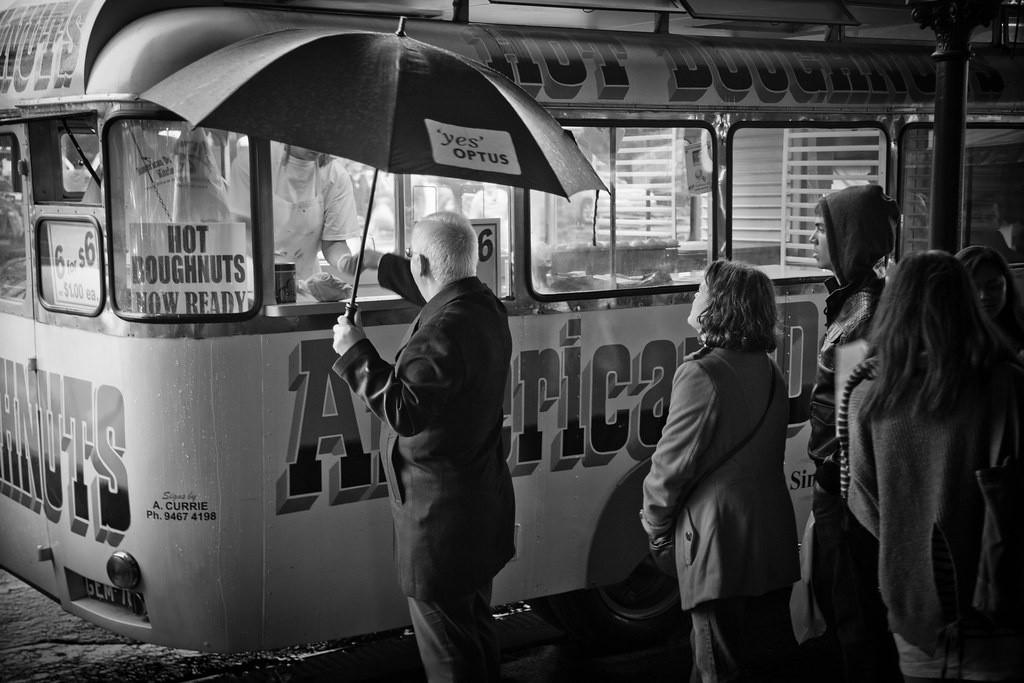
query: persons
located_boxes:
[331,211,516,683]
[227,130,362,300]
[641,185,1024,683]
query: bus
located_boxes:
[2,0,1024,656]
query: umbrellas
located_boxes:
[137,15,613,325]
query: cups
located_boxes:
[274,261,296,306]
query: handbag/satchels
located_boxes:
[972,359,1024,623]
[648,530,675,578]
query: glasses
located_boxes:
[405,247,416,257]
